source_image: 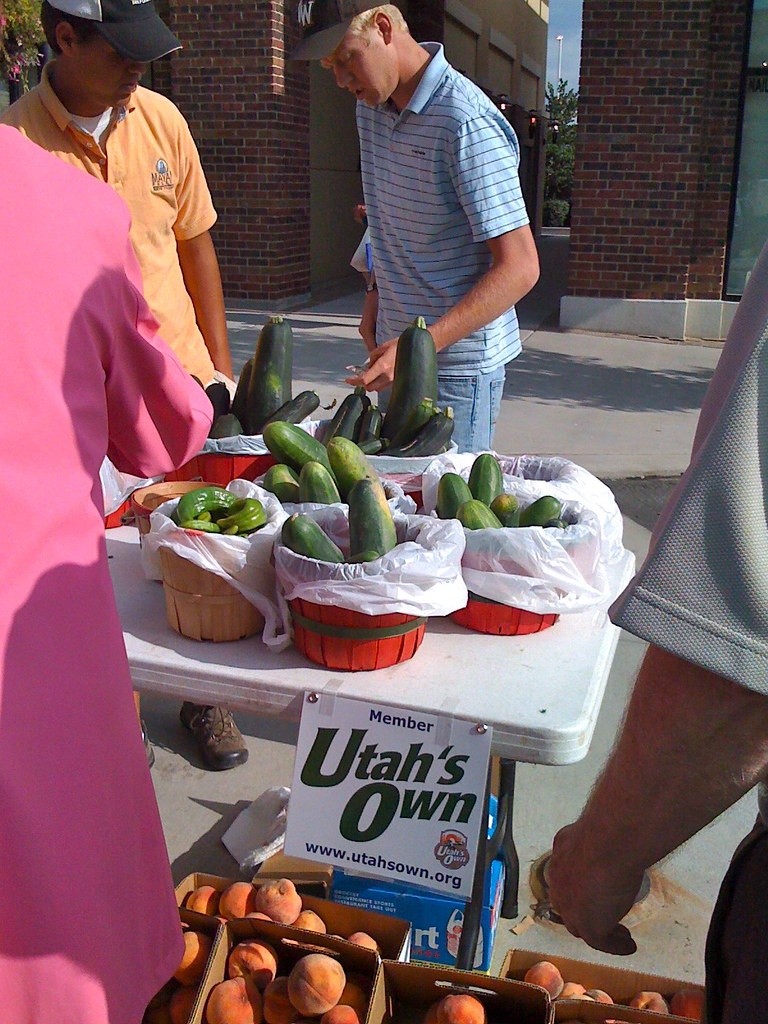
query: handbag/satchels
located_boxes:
[100,395,636,651]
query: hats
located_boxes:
[293,0,390,62]
[48,0,184,64]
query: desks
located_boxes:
[100,526,637,921]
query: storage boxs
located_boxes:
[169,848,707,1024]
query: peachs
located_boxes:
[140,878,706,1024]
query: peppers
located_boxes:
[171,487,267,537]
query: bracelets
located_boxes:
[365,282,377,295]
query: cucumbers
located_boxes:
[281,478,397,565]
[262,422,385,504]
[434,454,573,530]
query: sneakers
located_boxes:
[180,701,247,771]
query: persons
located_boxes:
[1,0,250,772]
[547,243,768,1024]
[0,125,213,1024]
[291,0,540,453]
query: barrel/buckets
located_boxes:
[130,416,596,672]
